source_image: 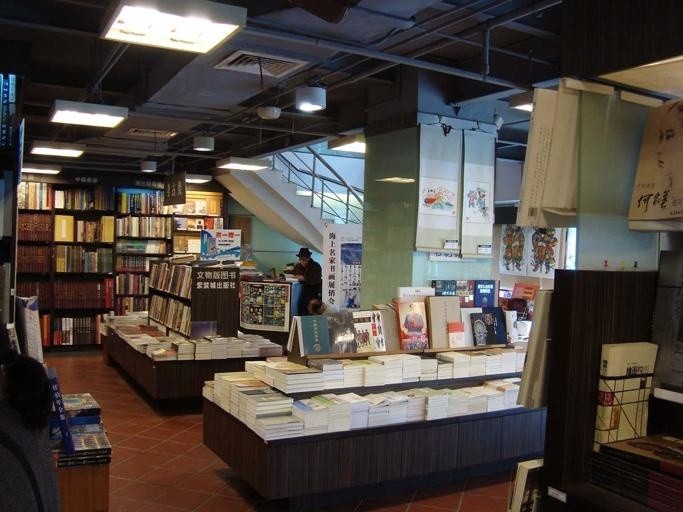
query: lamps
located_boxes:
[140,125,215,173]
[295,69,327,112]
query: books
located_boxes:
[17,182,114,345]
[1,73,25,354]
[202,280,534,441]
[589,434,682,511]
[511,458,543,511]
[108,311,282,360]
[173,198,223,252]
[6,296,112,466]
[590,342,657,452]
[149,264,192,336]
[171,255,263,276]
[516,289,550,405]
[115,193,172,315]
[281,273,298,281]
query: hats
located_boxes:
[295,248,312,257]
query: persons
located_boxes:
[1,347,61,511]
[290,249,321,315]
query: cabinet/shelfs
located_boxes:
[202,278,547,507]
[102,260,289,419]
[9,175,233,349]
